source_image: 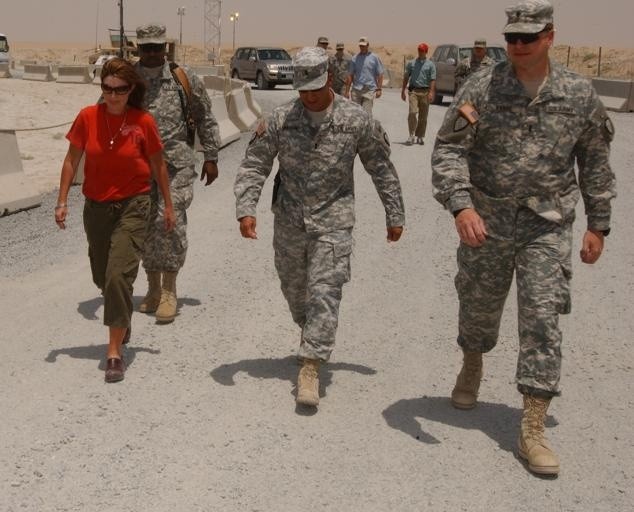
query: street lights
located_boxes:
[176,4,186,45]
[229,12,239,58]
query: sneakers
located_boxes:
[407,137,415,144]
[417,137,423,145]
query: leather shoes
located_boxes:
[106,358,124,382]
[123,326,131,345]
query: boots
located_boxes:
[517,393,559,475]
[451,352,483,408]
[297,358,320,406]
[138,271,162,313]
[156,271,177,322]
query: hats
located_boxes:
[418,44,428,53]
[337,44,345,49]
[359,39,369,46]
[318,37,328,44]
[293,46,328,90]
[136,24,166,44]
[473,40,486,49]
[502,0,554,34]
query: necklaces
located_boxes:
[103,108,127,150]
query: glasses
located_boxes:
[140,44,165,51]
[101,83,132,95]
[505,34,539,44]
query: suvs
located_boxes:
[231,46,300,91]
[423,40,507,103]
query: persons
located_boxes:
[454,39,496,95]
[315,36,346,95]
[400,42,436,147]
[231,44,407,408]
[95,23,221,326]
[50,58,178,386]
[351,37,385,119]
[430,0,618,479]
[332,40,355,101]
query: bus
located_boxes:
[0,33,11,65]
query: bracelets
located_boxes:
[377,89,381,92]
[599,226,611,237]
[54,202,67,210]
[205,159,218,163]
